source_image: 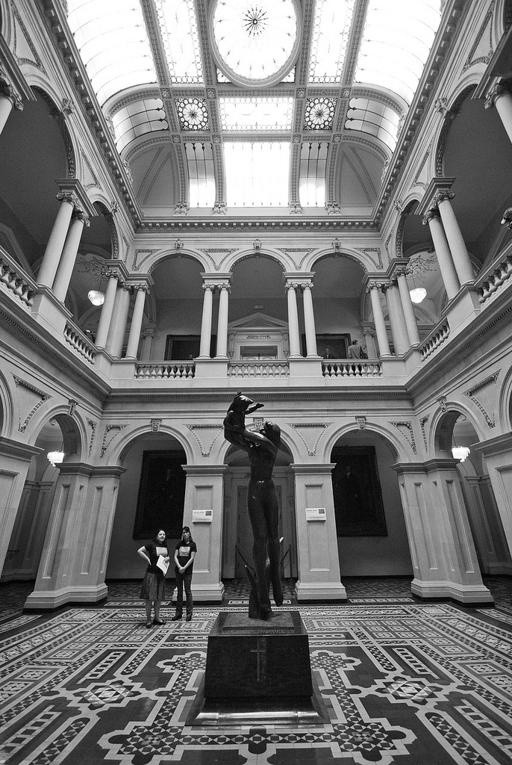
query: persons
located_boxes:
[136,527,171,629]
[222,391,265,448]
[222,412,285,622]
[321,346,337,377]
[346,337,364,376]
[170,526,198,622]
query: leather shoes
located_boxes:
[147,619,166,628]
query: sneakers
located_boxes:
[172,613,192,621]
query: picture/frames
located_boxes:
[331,446,388,538]
[164,335,216,360]
[302,333,351,359]
[133,450,186,540]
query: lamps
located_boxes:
[88,278,106,307]
[409,275,427,304]
[47,441,66,469]
[451,432,471,463]
